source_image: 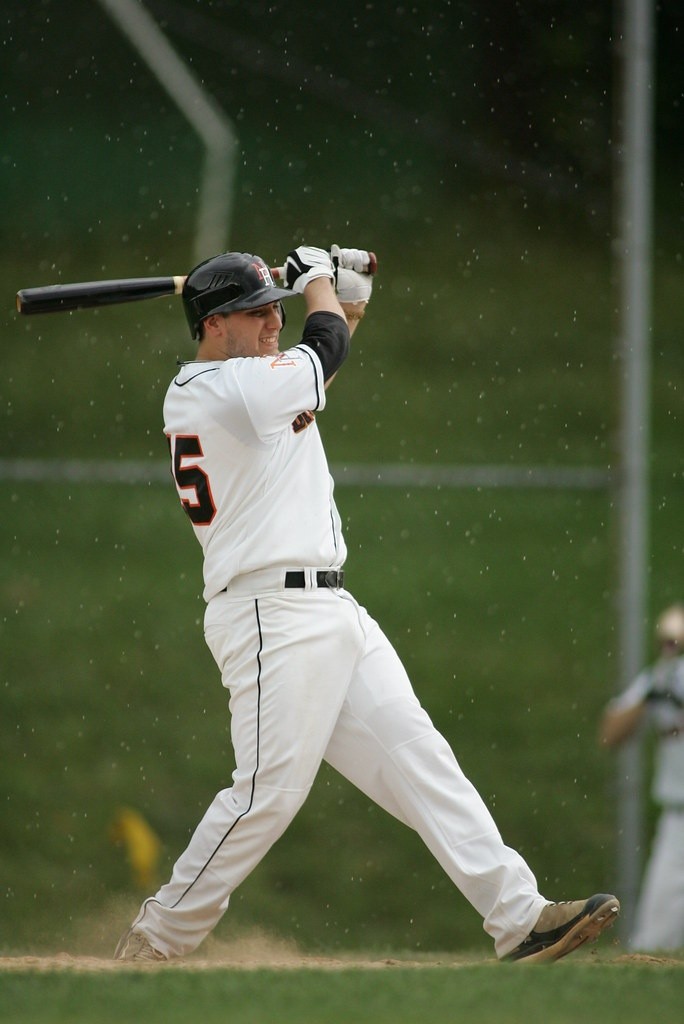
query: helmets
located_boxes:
[182,252,299,340]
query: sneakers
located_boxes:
[113,926,167,964]
[499,892,621,962]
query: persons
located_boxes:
[599,602,683,957]
[118,243,621,966]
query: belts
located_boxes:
[221,570,344,591]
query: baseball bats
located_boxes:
[16,255,377,315]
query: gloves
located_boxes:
[284,246,336,293]
[330,244,377,305]
